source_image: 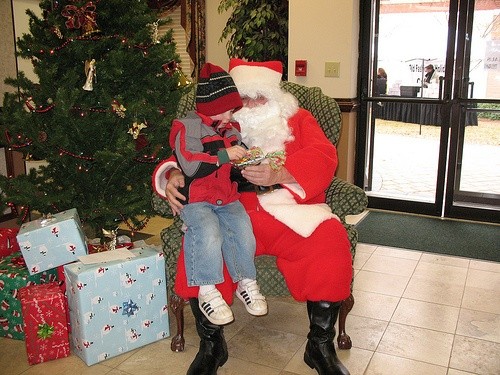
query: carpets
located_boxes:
[355,211,500,262]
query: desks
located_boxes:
[373,94,479,127]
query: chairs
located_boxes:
[151,81,370,354]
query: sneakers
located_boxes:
[235,279,268,316]
[198,288,234,325]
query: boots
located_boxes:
[185,297,229,375]
[303,299,351,375]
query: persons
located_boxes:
[377,68,387,78]
[424,64,436,88]
[152,58,353,375]
[170,62,268,325]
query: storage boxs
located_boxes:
[0,207,170,367]
[400,86,419,97]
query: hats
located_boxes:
[196,62,244,116]
[229,58,283,99]
